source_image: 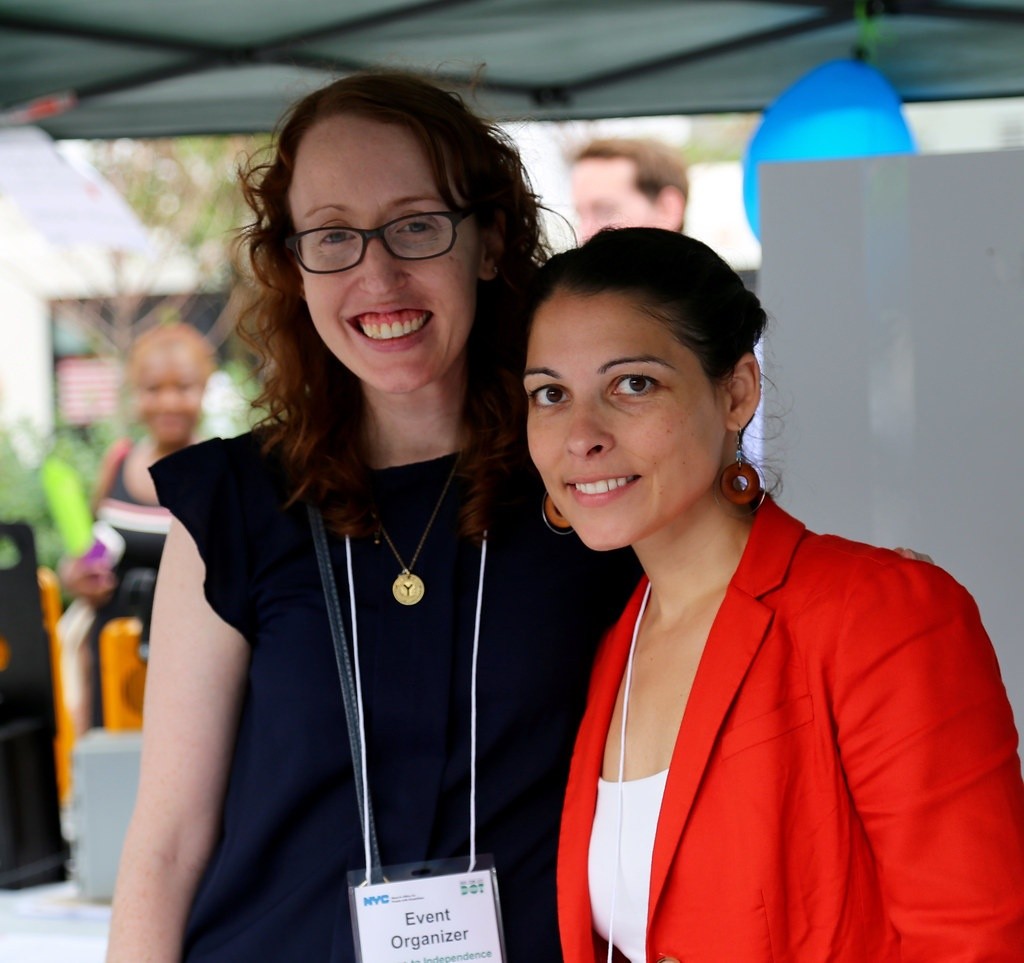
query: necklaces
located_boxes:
[374,452,455,606]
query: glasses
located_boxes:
[280,195,480,277]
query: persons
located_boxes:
[108,70,645,963]
[55,323,218,728]
[522,229,1024,963]
[571,140,761,465]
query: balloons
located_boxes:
[744,61,915,240]
